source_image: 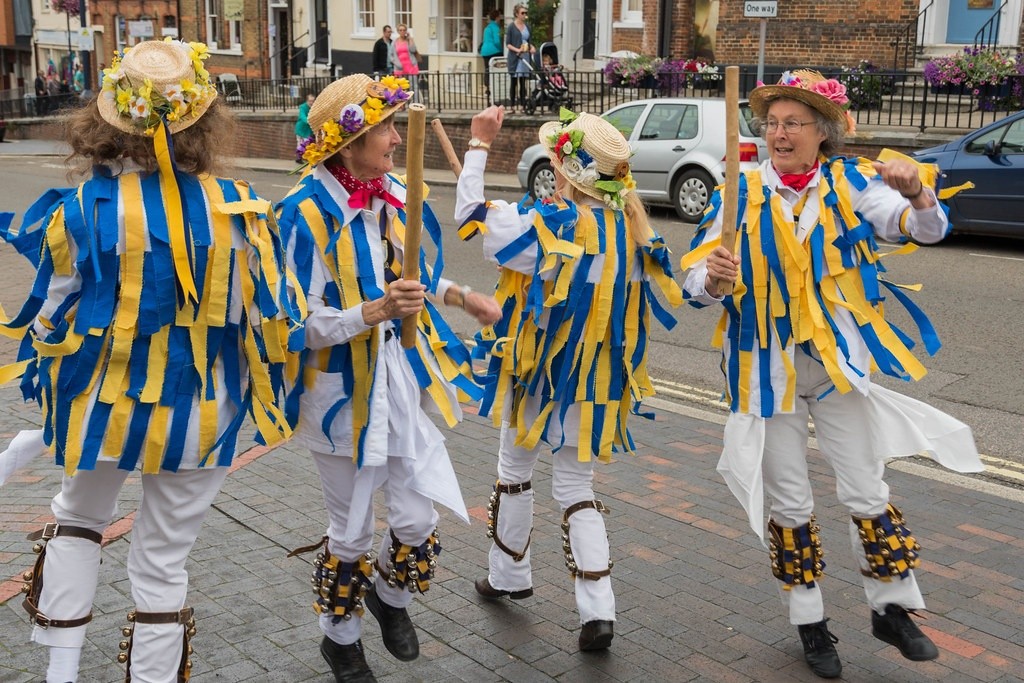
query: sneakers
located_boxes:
[872,604,938,662]
[798,617,842,678]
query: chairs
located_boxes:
[651,120,678,139]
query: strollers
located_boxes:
[515,41,573,115]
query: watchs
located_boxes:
[468,139,490,151]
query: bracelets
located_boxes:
[901,180,922,198]
[459,287,473,310]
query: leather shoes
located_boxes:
[578,618,615,655]
[364,584,420,662]
[321,635,377,683]
[474,575,535,599]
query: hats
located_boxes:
[294,72,416,167]
[750,68,857,143]
[538,111,639,207]
[95,37,219,138]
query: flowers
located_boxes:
[604,55,723,96]
[833,58,898,112]
[100,34,218,137]
[285,75,410,175]
[52,0,80,17]
[547,106,638,215]
[922,42,1024,96]
[756,70,853,114]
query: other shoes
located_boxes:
[397,107,406,113]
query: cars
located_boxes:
[517,97,770,225]
[906,110,1024,240]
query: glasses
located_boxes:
[399,29,406,32]
[760,117,820,134]
[518,11,528,15]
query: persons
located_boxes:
[453,106,685,650]
[372,24,418,112]
[35,64,85,115]
[506,3,535,113]
[271,73,503,683]
[478,11,504,85]
[681,71,985,678]
[295,94,315,164]
[543,55,568,89]
[0,38,288,683]
[98,63,106,89]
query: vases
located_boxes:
[930,75,1013,97]
[611,72,718,91]
[977,74,1024,111]
